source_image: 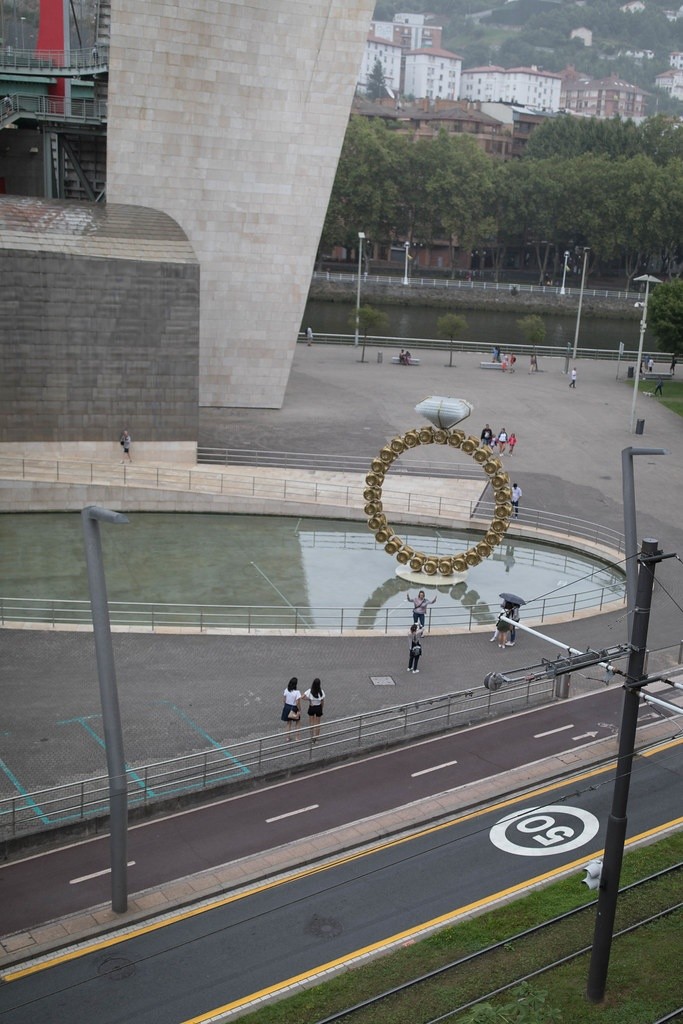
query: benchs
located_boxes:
[644,371,672,380]
[480,362,508,370]
[392,357,419,365]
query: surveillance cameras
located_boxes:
[634,303,639,308]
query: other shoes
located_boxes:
[287,738,292,742]
[511,641,516,645]
[502,645,505,648]
[420,633,424,638]
[497,643,502,648]
[490,638,495,641]
[295,738,301,742]
[505,642,513,647]
[413,670,419,673]
[406,667,413,671]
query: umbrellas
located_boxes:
[499,593,526,606]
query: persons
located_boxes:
[92,45,98,65]
[481,424,516,457]
[512,483,522,519]
[544,273,548,279]
[640,281,645,293]
[492,347,516,373]
[121,431,132,463]
[280,677,301,743]
[645,353,654,372]
[399,349,411,365]
[407,591,437,637]
[301,678,325,736]
[490,600,520,648]
[654,376,664,396]
[670,356,676,375]
[407,624,425,674]
[307,326,313,346]
[569,367,576,388]
[528,355,535,374]
[4,94,12,114]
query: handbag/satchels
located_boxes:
[412,646,421,656]
[288,710,300,720]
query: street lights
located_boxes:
[573,246,592,359]
[354,231,366,348]
[403,241,411,285]
[584,443,677,1005]
[80,505,132,913]
[630,274,664,434]
[560,250,570,294]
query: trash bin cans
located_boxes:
[378,351,383,363]
[627,366,634,378]
[556,672,572,699]
[636,419,645,435]
